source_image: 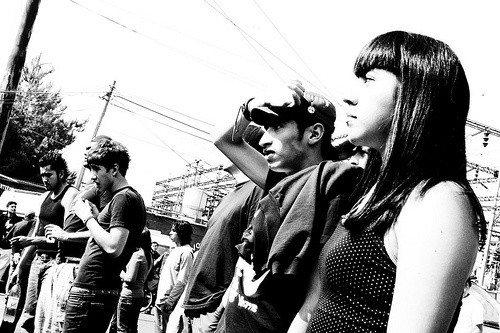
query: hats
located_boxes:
[250,91,336,132]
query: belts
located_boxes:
[38,254,55,261]
[59,257,80,264]
[69,286,118,295]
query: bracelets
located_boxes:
[241,98,256,122]
[83,215,95,225]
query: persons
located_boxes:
[203,78,364,333]
[108,227,169,333]
[0,150,80,333]
[166,124,266,333]
[60,135,147,333]
[285,31,488,333]
[33,135,112,333]
[155,219,194,333]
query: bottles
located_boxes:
[4,284,21,322]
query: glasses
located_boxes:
[83,164,104,170]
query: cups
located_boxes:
[46,226,55,243]
